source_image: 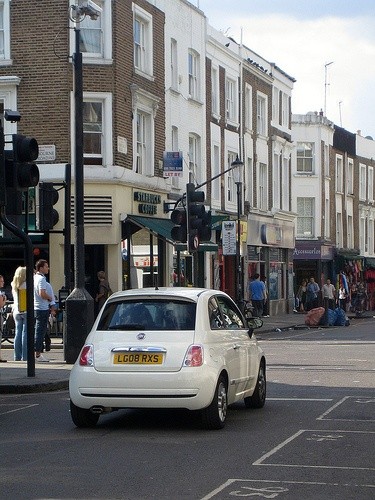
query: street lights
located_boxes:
[230,154,245,300]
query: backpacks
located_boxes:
[100,284,113,298]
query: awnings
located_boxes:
[125,215,219,251]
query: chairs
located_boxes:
[128,302,154,325]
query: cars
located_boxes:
[69,286,268,429]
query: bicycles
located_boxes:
[0,298,17,343]
[219,298,255,320]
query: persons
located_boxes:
[248,272,267,316]
[337,281,370,312]
[11,266,28,360]
[0,275,7,362]
[296,278,337,311]
[95,271,109,310]
[32,259,54,361]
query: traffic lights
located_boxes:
[185,183,208,254]
[12,134,41,188]
[0,158,24,217]
[171,208,187,244]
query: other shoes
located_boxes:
[35,354,49,362]
